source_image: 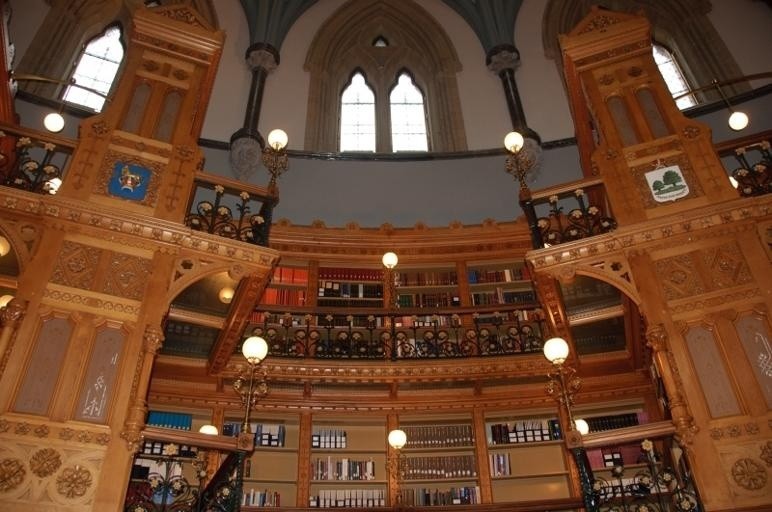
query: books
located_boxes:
[257,287,307,306]
[317,267,385,308]
[472,311,539,324]
[310,454,375,480]
[468,266,536,306]
[394,314,464,328]
[220,453,254,477]
[238,487,281,506]
[271,267,309,286]
[311,427,347,448]
[219,420,286,447]
[247,311,318,327]
[309,486,386,508]
[319,315,394,328]
[396,271,460,308]
[582,409,649,436]
[398,423,482,508]
[484,418,564,479]
[126,408,192,507]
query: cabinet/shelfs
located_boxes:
[128,403,216,507]
[303,407,396,510]
[385,264,473,355]
[216,404,304,508]
[237,260,313,356]
[562,396,683,501]
[460,259,549,357]
[477,403,579,505]
[391,407,490,505]
[312,262,392,356]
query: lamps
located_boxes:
[503,131,536,191]
[542,337,584,431]
[388,428,408,450]
[230,335,270,432]
[575,418,590,437]
[200,424,218,440]
[262,129,288,187]
[381,252,399,270]
